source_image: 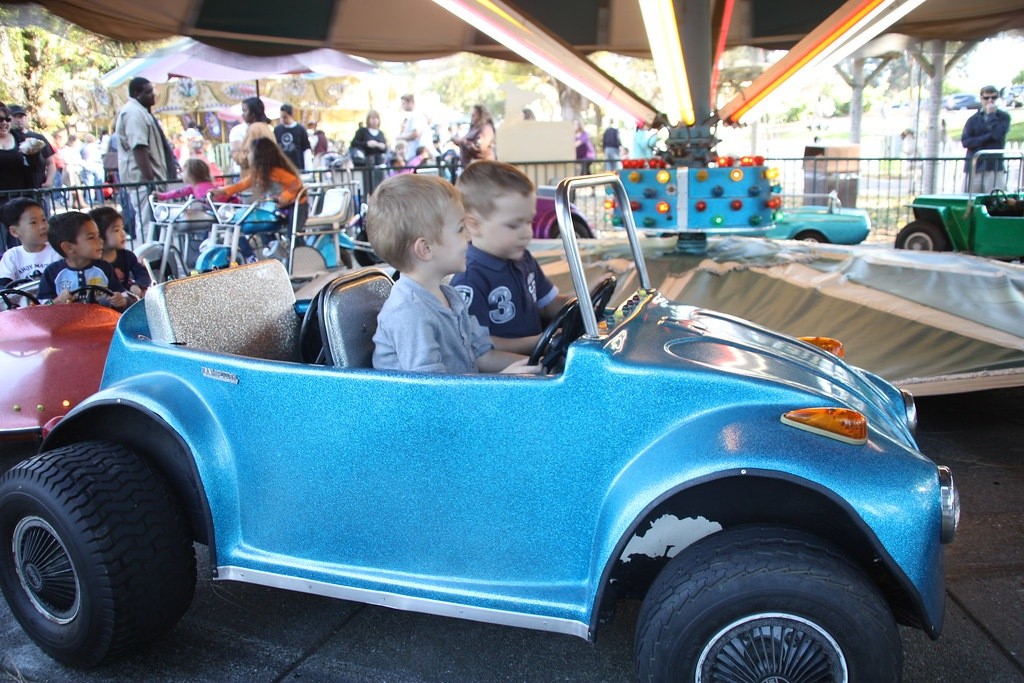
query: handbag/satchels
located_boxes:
[349,147,366,166]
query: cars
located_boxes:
[941,86,1024,111]
[0,277,124,432]
[767,191,871,245]
[895,148,1024,262]
[1,172,961,683]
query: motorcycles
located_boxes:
[131,181,361,290]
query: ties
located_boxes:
[149,111,177,178]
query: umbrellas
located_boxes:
[98,38,380,127]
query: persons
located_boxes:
[0,78,668,314]
[365,174,557,377]
[447,161,615,371]
[960,86,1011,195]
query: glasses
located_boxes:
[13,114,24,117]
[983,96,997,101]
[0,117,11,122]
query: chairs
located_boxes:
[144,259,301,363]
[305,188,351,226]
[315,268,395,368]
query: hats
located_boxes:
[9,104,27,116]
